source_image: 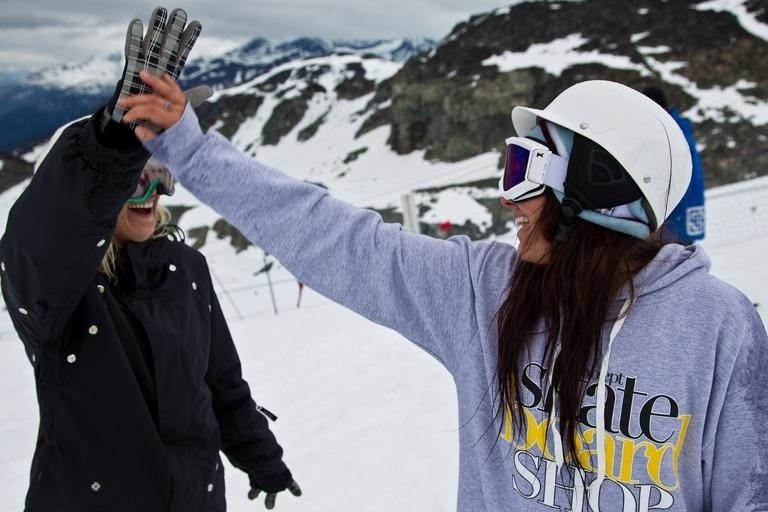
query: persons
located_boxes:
[2,9,302,509]
[116,69,768,511]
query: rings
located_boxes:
[162,102,172,113]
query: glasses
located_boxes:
[125,169,175,202]
[498,136,556,202]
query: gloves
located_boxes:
[105,7,214,133]
[248,460,302,509]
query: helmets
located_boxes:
[511,79,693,233]
